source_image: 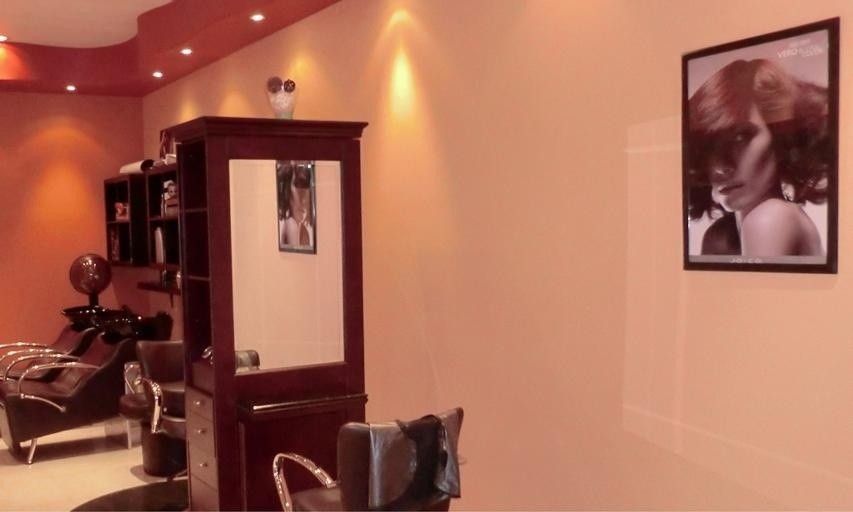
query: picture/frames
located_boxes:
[680,16,838,273]
[275,160,317,255]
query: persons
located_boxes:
[280,161,315,250]
[688,58,828,257]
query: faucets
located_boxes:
[120,305,130,314]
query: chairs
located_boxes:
[121,340,184,475]
[274,408,464,512]
[1,322,93,383]
[0,334,128,465]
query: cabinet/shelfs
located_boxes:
[105,163,180,294]
[167,116,369,512]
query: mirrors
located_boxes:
[209,135,365,395]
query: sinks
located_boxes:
[81,310,139,337]
[60,306,101,324]
